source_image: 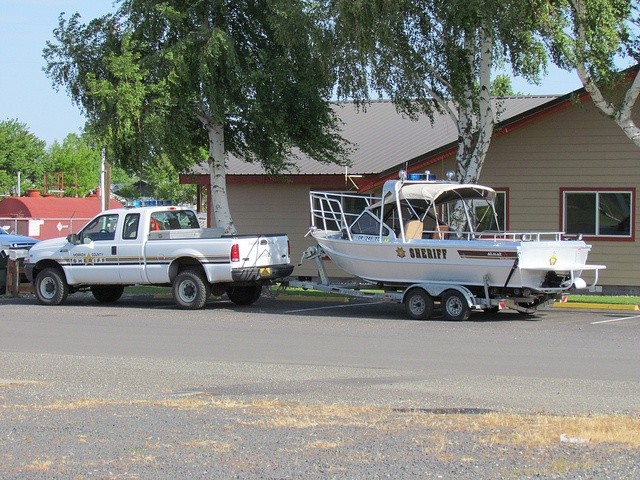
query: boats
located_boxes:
[304,160,607,293]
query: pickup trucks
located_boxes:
[23,205,295,310]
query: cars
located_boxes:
[0,227,40,251]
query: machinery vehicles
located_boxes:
[265,280,568,322]
